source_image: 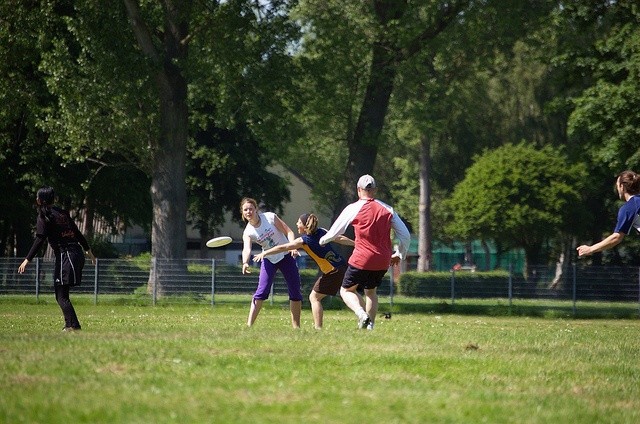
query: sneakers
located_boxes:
[367,320,374,329]
[358,313,369,329]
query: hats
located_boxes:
[357,174,376,190]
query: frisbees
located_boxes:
[206,236,234,248]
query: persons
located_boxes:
[17,184,96,332]
[576,169,640,258]
[251,213,355,327]
[318,175,410,329]
[239,197,302,327]
